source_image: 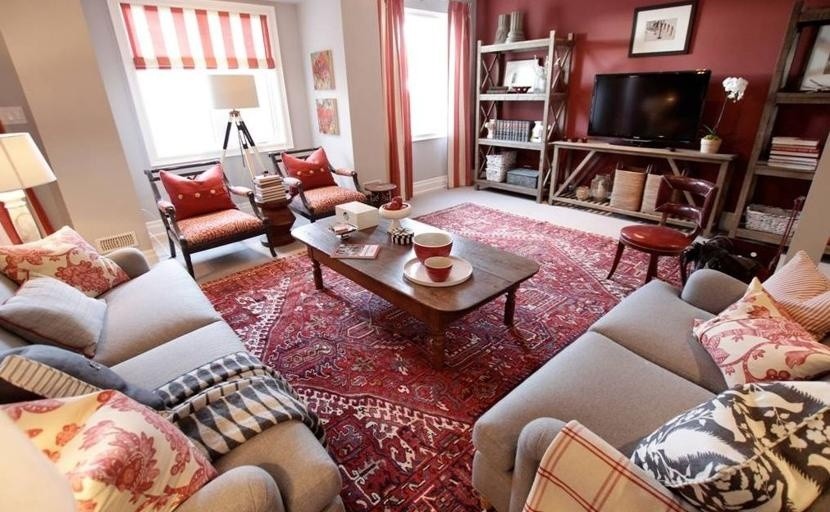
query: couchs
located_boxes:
[0,225,351,512]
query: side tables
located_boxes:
[253,192,297,247]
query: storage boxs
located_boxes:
[507,168,538,189]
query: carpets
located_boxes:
[200,203,704,512]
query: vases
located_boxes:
[700,139,722,155]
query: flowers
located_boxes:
[702,77,747,139]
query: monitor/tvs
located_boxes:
[587,69,712,150]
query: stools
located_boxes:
[365,181,397,208]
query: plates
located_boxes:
[403,255,472,288]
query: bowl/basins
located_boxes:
[413,233,453,270]
[424,256,453,282]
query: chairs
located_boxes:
[269,146,367,222]
[144,160,276,279]
[606,176,719,288]
[472,269,830,512]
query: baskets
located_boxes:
[610,160,653,212]
[639,160,689,216]
[745,204,801,236]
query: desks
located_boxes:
[548,140,737,239]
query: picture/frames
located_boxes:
[628,0,698,56]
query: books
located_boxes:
[489,86,508,90]
[329,242,381,260]
[493,119,532,143]
[487,89,509,94]
[767,136,821,171]
[251,174,288,204]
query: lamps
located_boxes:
[1,132,57,243]
[209,74,267,178]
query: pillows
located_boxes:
[281,147,338,196]
[160,163,240,222]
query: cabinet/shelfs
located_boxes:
[474,30,575,203]
[729,0,829,254]
